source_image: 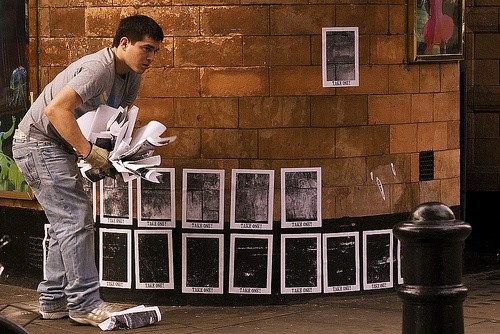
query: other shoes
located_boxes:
[39,303,70,320]
[69,303,116,327]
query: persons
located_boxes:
[12,15,164,328]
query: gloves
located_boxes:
[82,140,119,180]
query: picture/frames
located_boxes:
[407,0,464,65]
